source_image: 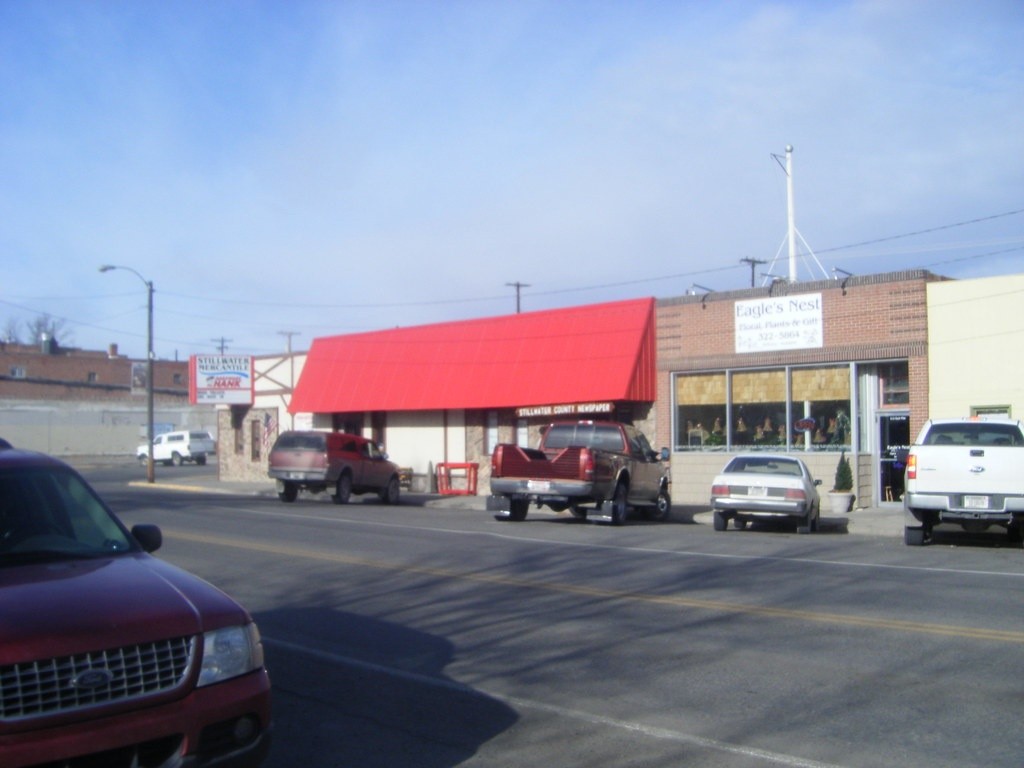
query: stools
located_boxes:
[885,486,894,502]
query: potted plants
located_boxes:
[827,447,854,513]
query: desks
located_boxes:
[880,458,898,462]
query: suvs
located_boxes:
[136,430,216,467]
[267,430,401,505]
[895,416,1024,546]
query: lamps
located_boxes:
[832,267,852,281]
[686,283,713,295]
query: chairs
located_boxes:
[992,437,1012,446]
[934,434,953,444]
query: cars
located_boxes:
[708,452,823,535]
[0,436,274,768]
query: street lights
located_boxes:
[505,282,530,314]
[97,262,155,481]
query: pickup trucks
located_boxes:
[484,418,673,527]
